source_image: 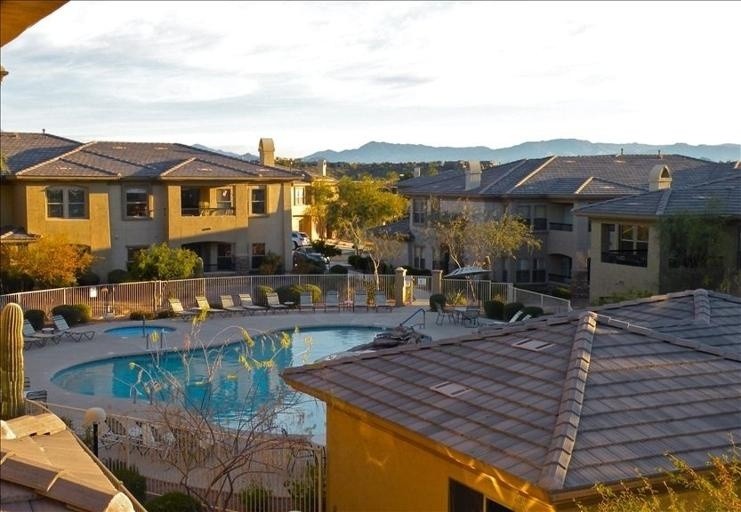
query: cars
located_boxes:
[292,231,331,266]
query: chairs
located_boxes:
[168,289,392,321]
[495,310,532,325]
[22,314,95,350]
[435,301,480,327]
[101,415,239,464]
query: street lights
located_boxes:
[85,407,106,458]
